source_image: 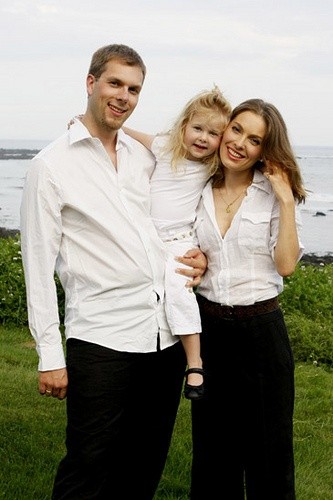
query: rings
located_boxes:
[46,389,52,395]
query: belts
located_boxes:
[195,295,280,321]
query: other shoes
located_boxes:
[183,368,207,401]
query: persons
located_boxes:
[188,98,307,500]
[20,43,208,500]
[65,83,232,401]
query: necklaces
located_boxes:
[216,171,254,213]
